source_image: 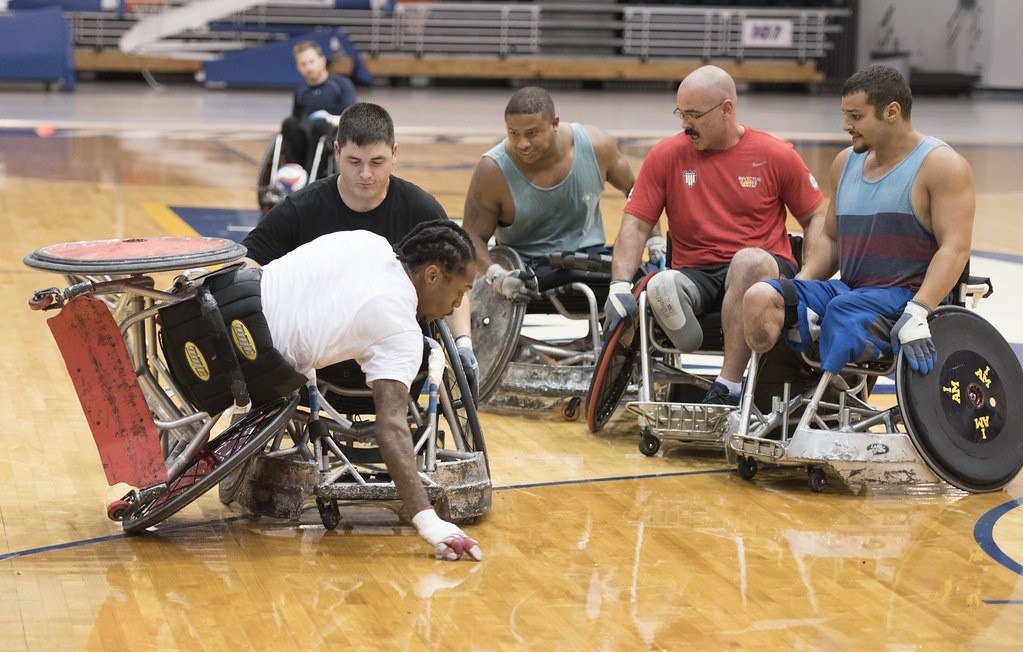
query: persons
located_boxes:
[603,65,825,406]
[274,41,358,195]
[240,103,450,412]
[741,66,977,376]
[459,86,666,315]
[155,220,486,561]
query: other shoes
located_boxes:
[273,163,307,195]
[701,382,740,405]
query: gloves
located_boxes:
[412,505,483,561]
[601,279,637,341]
[486,264,538,304]
[455,336,479,381]
[890,301,937,375]
[311,109,339,126]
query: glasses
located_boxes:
[673,106,724,121]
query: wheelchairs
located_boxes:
[257,130,339,216]
[24,236,492,533]
[468,246,1023,493]
